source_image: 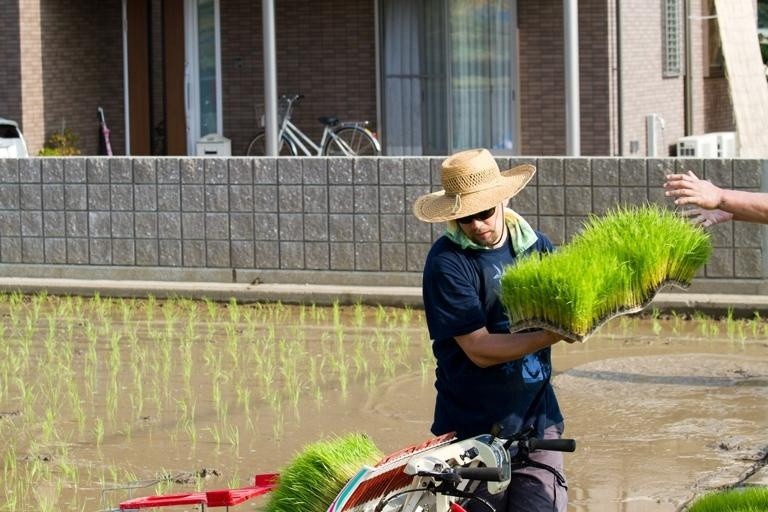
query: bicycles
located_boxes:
[246,94,382,157]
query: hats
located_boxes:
[414,148,537,223]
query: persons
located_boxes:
[412,146,569,511]
[662,169,768,229]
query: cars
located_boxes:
[0,117,28,158]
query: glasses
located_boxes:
[458,207,495,224]
